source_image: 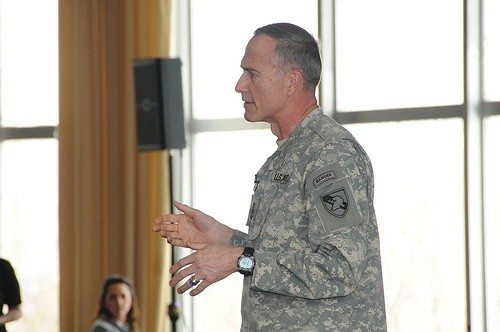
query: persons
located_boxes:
[153,22,386,332]
[91,274,137,332]
[0,258,22,331]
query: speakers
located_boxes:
[132,57,186,151]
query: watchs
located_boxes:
[237,247,256,274]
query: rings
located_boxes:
[189,276,199,286]
